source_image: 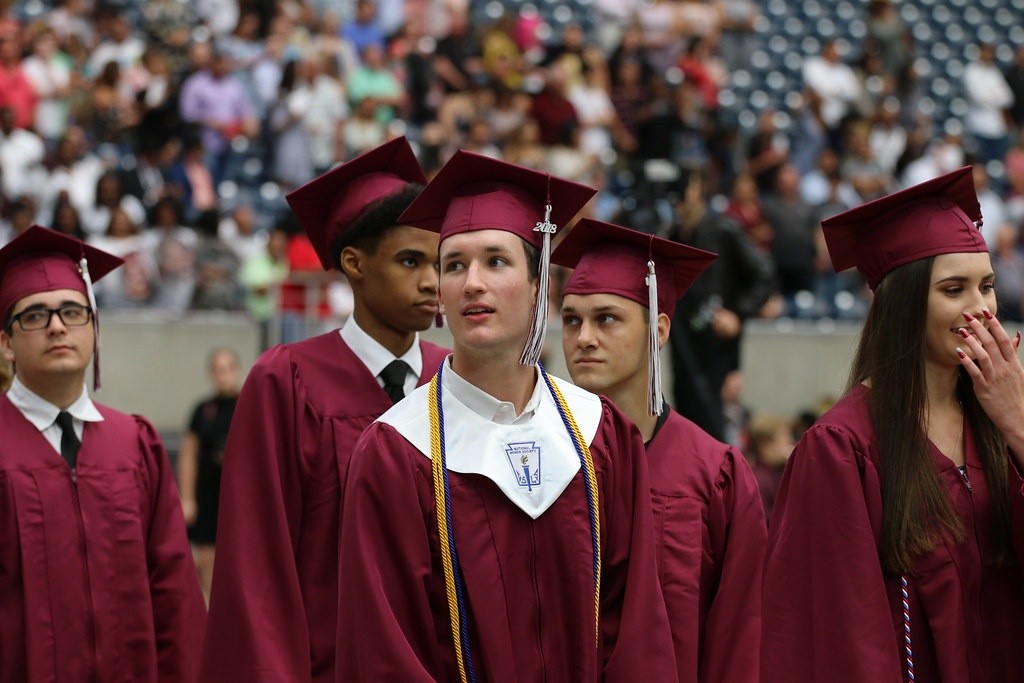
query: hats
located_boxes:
[286,136,429,271]
[396,150,598,368]
[821,165,989,289]
[0,225,125,392]
[550,218,719,417]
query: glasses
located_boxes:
[5,305,92,331]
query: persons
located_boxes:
[177,135,770,683]
[0,0,1024,325]
[0,222,207,683]
[758,165,1024,682]
